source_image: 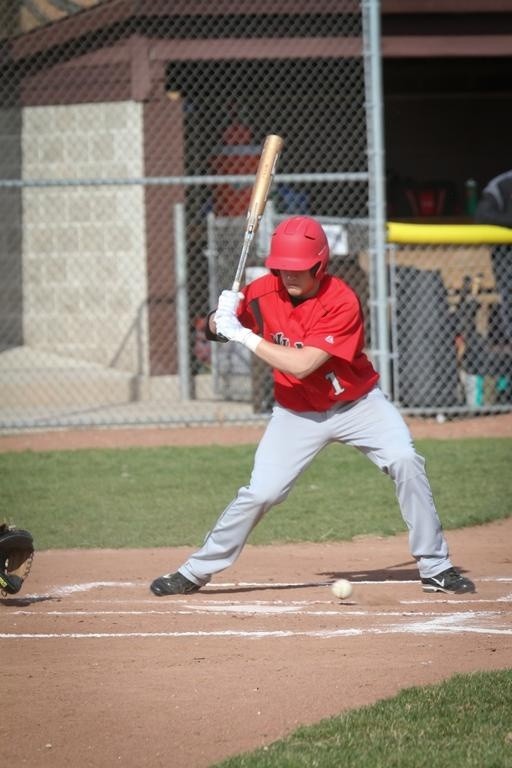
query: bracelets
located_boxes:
[245,331,261,352]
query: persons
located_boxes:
[149,215,475,596]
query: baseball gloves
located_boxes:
[1,523,34,596]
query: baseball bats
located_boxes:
[216,134,283,341]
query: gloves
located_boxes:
[216,290,245,315]
[216,315,252,344]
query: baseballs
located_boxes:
[332,578,352,599]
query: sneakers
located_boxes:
[150,570,201,595]
[422,567,476,594]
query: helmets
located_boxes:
[265,216,329,280]
